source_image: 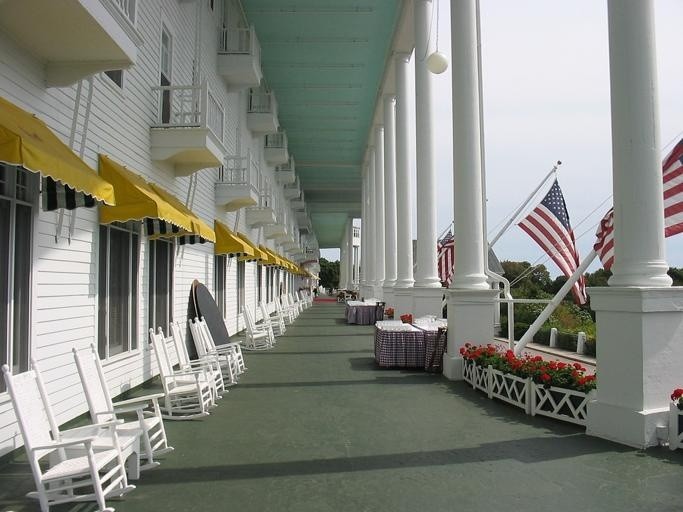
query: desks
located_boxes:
[372,319,447,365]
[346,299,383,325]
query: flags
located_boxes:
[516,178,588,304]
[437,229,454,286]
[593,137,683,272]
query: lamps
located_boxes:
[426,2,449,75]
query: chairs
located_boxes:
[72,343,174,474]
[149,326,218,421]
[2,357,137,511]
[197,318,248,376]
[187,318,243,387]
[169,321,229,399]
[241,289,312,351]
